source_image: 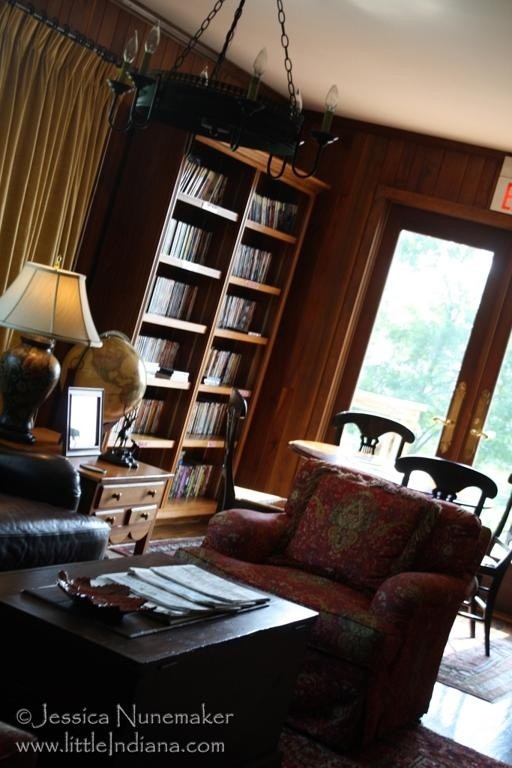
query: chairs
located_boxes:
[0,450,110,571]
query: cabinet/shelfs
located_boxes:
[75,100,334,527]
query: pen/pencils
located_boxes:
[169,614,214,625]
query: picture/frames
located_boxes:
[62,383,106,458]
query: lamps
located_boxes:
[0,257,105,445]
[103,1,347,182]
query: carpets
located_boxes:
[278,724,511,767]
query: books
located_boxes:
[94,562,270,626]
[110,156,297,501]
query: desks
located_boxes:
[35,427,176,556]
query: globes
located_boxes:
[56,330,147,446]
